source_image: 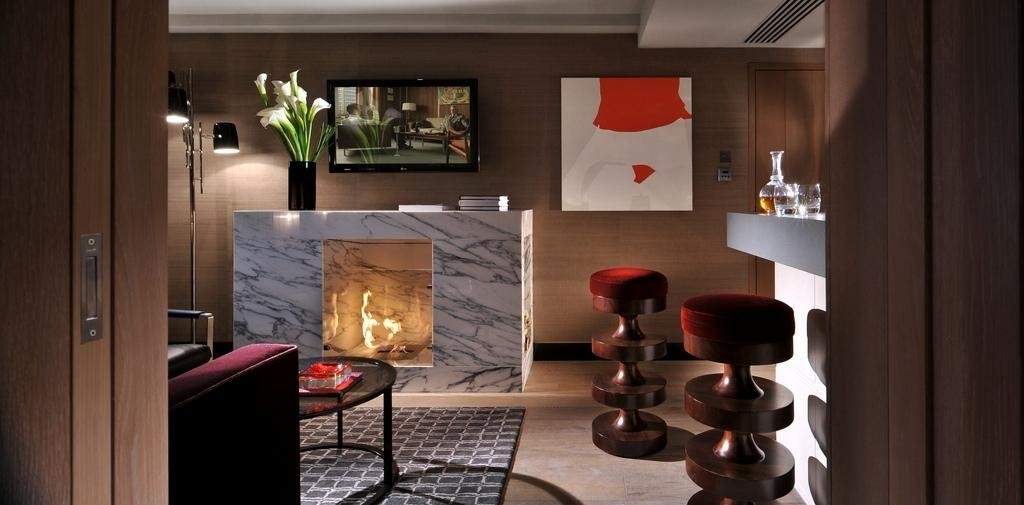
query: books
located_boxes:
[397,201,454,211]
[457,205,509,212]
[457,199,510,205]
[458,192,509,199]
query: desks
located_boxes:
[400,131,453,153]
[299,356,396,505]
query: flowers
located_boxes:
[341,101,403,166]
[253,63,336,165]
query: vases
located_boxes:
[286,162,316,210]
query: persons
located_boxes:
[438,103,467,133]
[341,100,369,122]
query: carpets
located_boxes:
[298,405,528,505]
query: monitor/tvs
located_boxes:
[326,76,480,173]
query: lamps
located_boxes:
[167,61,240,342]
[402,101,417,122]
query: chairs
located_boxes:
[446,124,470,163]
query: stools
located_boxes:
[678,292,796,505]
[589,266,669,459]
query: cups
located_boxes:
[774,183,822,218]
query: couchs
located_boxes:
[407,117,470,139]
[168,343,301,505]
[168,303,215,378]
[338,124,392,156]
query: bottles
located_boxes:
[758,150,789,216]
